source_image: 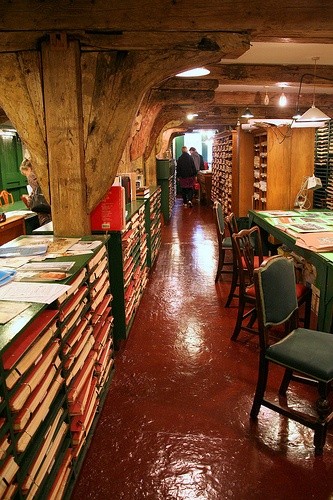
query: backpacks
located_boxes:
[199,155,205,170]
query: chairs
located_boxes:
[214,200,333,454]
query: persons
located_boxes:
[177,146,197,207]
[189,147,204,198]
[20,159,51,227]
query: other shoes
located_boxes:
[189,201,193,207]
[183,203,188,208]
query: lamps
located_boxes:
[241,105,254,118]
[297,57,331,123]
[293,73,333,118]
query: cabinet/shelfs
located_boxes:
[212,119,332,219]
[0,161,176,500]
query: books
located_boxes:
[136,186,150,196]
[169,179,175,218]
[90,172,136,230]
[253,141,267,202]
[121,206,148,326]
[314,126,333,210]
[0,246,116,500]
[211,137,232,213]
[150,188,162,260]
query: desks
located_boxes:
[248,209,332,333]
